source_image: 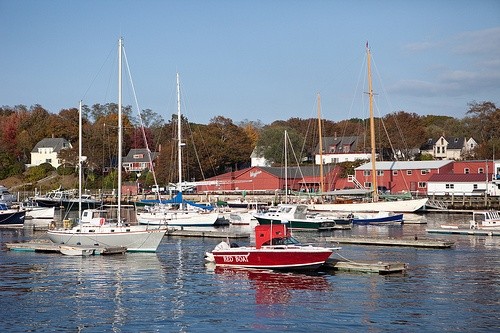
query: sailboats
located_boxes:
[0,39,429,238]
[46,33,168,252]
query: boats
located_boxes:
[202,219,342,270]
[425,207,500,236]
